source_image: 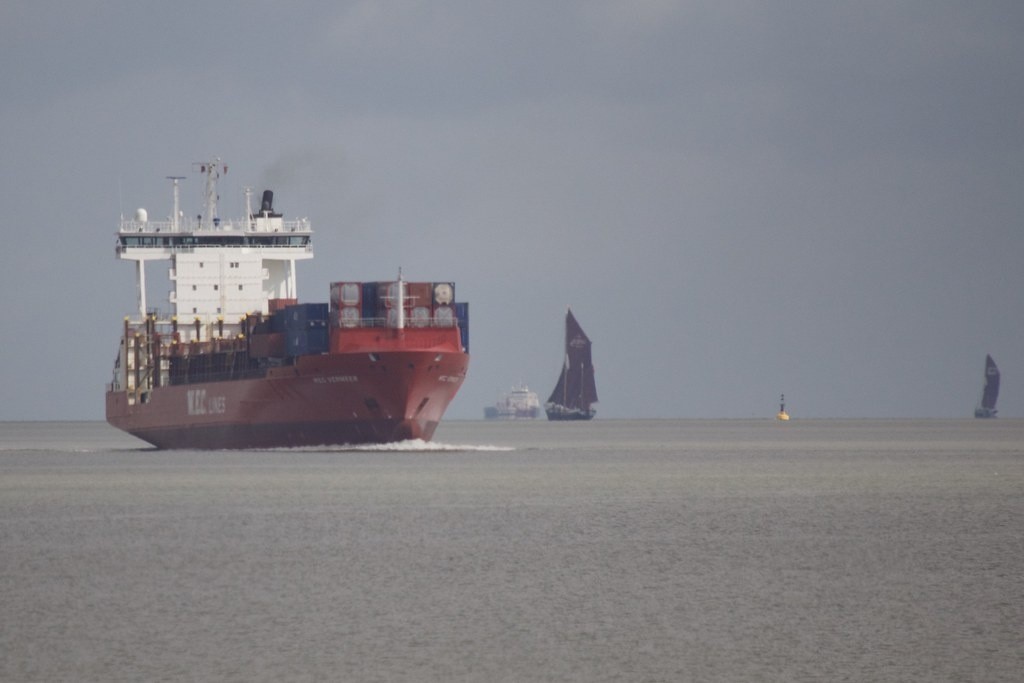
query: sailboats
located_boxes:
[972,351,1001,418]
[543,307,598,420]
[777,391,789,421]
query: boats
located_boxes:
[494,377,541,419]
[104,155,470,450]
